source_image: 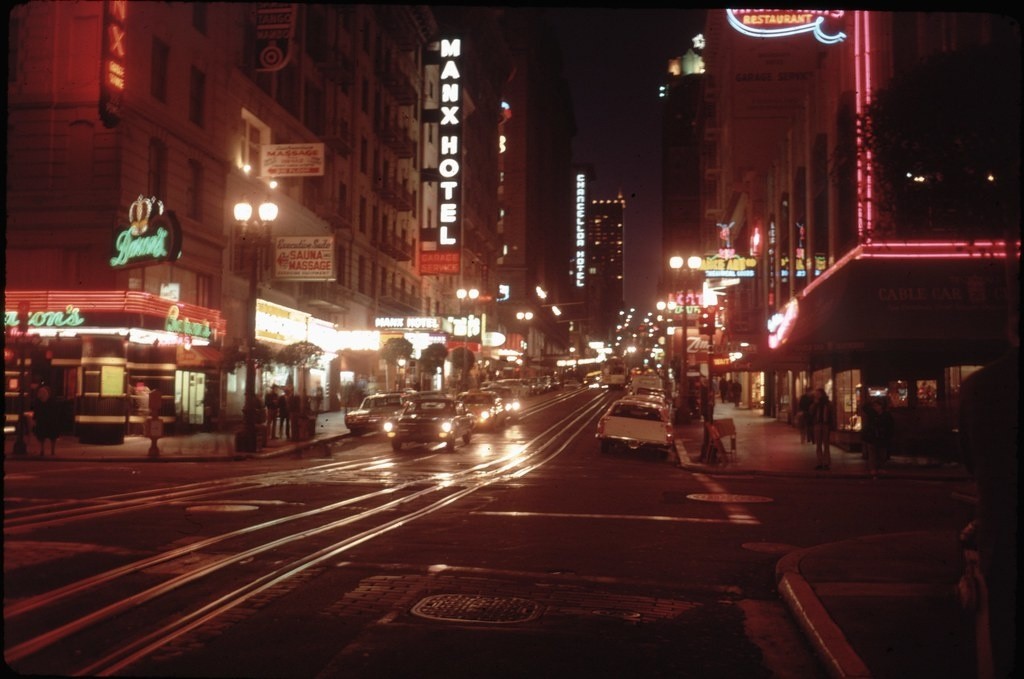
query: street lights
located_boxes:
[669,255,703,428]
[517,312,533,378]
[232,200,280,454]
[456,287,479,392]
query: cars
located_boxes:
[343,393,408,436]
[453,371,568,432]
[383,398,476,453]
[595,356,674,460]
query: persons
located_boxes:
[718,377,742,406]
[264,384,294,440]
[800,384,833,470]
[342,371,379,415]
[956,300,1023,679]
[476,367,496,382]
[448,375,457,395]
[312,379,324,400]
[861,398,894,469]
[412,380,421,391]
[32,386,58,456]
